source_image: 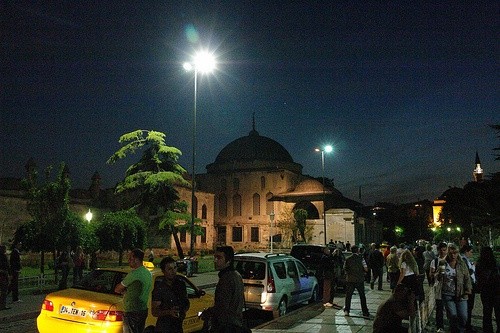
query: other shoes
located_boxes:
[323,302,333,307]
[1,306,12,311]
[16,300,22,303]
[344,310,349,316]
[363,314,373,319]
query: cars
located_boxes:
[36,261,215,333]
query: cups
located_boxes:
[440,266,445,274]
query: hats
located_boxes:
[380,243,389,247]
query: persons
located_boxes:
[59,246,106,288]
[148,251,154,262]
[330,239,351,251]
[429,237,477,333]
[0,238,23,310]
[114,249,151,333]
[373,283,415,333]
[360,240,434,312]
[151,256,189,333]
[320,247,339,307]
[475,246,500,333]
[343,246,371,316]
[199,246,243,333]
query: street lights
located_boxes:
[188,48,222,254]
[84,209,94,270]
[321,144,334,241]
[269,212,276,253]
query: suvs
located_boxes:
[290,244,351,294]
[233,252,321,319]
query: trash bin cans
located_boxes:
[177,257,198,275]
